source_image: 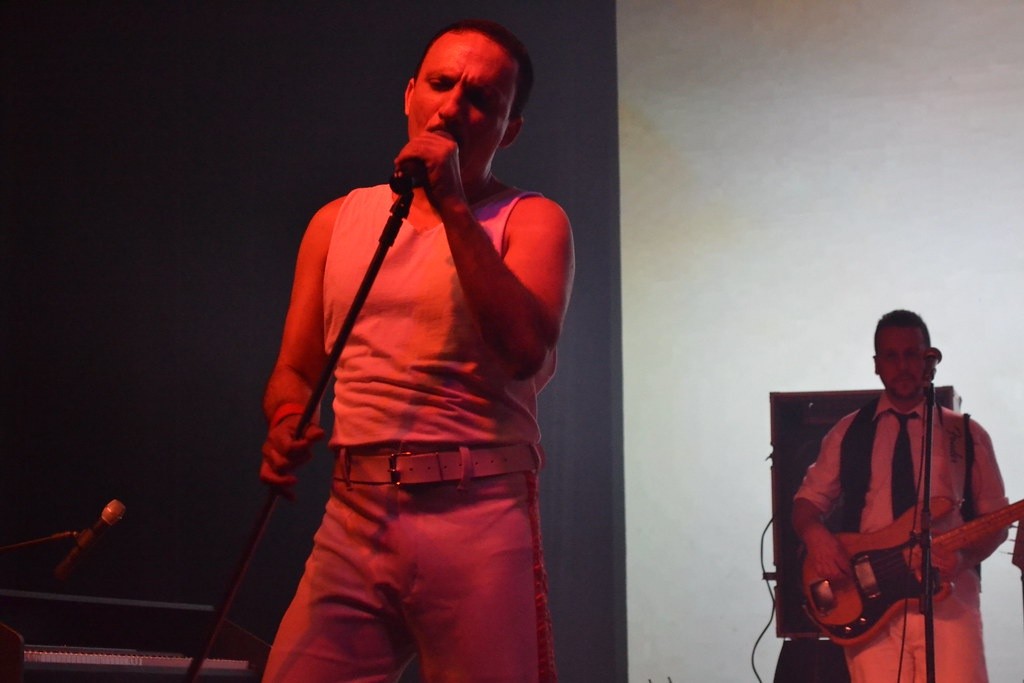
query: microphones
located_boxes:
[54,499,126,583]
[921,348,942,384]
[390,131,461,196]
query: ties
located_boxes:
[887,407,920,524]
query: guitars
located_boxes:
[802,496,1024,648]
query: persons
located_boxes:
[790,310,1010,683]
[261,21,575,683]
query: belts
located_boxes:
[331,442,548,489]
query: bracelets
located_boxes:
[267,400,311,430]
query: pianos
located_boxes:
[0,589,273,683]
